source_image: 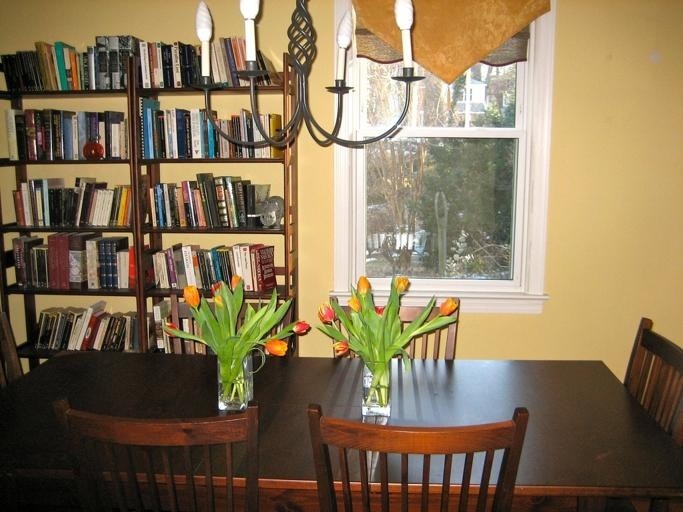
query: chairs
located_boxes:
[170,292,294,357]
[327,304,459,360]
[307,402,529,512]
[624,318,683,454]
[1,311,24,400]
[50,396,261,512]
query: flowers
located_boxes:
[315,276,462,408]
[160,276,312,407]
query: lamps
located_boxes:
[194,0,426,148]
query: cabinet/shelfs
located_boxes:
[0,54,153,376]
[132,52,301,357]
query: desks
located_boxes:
[0,350,683,512]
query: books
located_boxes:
[138,96,285,159]
[152,243,277,291]
[150,173,271,228]
[12,178,131,227]
[138,36,282,88]
[12,231,136,290]
[34,301,139,352]
[5,109,130,161]
[154,299,284,356]
[2,36,144,91]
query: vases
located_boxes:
[215,350,254,412]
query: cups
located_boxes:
[240,354,253,402]
[215,353,248,411]
